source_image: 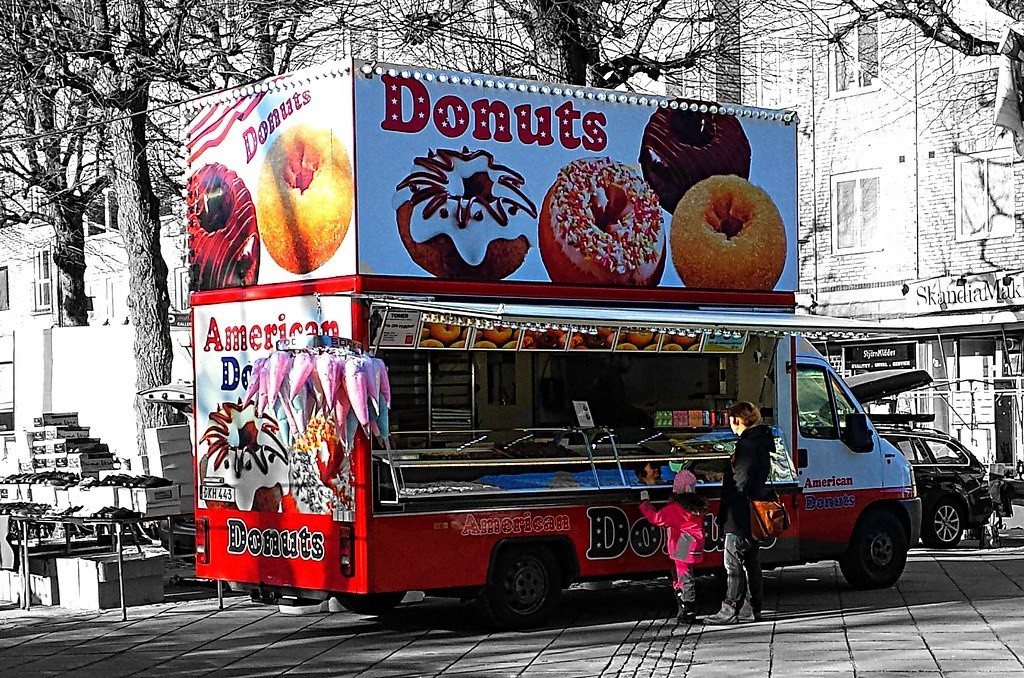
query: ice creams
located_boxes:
[243,351,391,440]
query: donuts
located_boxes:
[394,147,540,281]
[421,323,700,352]
[188,163,259,289]
[258,129,353,275]
[671,175,787,290]
[550,156,666,281]
[640,99,751,216]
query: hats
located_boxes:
[672,469,696,494]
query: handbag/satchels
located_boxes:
[750,500,791,541]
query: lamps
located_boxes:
[901,275,944,296]
[956,268,1003,286]
[1002,271,1024,286]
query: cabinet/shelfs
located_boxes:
[373,423,800,505]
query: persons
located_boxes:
[638,469,710,624]
[703,401,777,626]
[563,364,655,429]
[635,462,674,485]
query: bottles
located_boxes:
[726,400,733,410]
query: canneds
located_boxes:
[703,410,729,426]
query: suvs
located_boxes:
[874,424,994,549]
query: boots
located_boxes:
[674,588,696,624]
[737,597,762,621]
[703,601,739,625]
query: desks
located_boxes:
[10,514,194,622]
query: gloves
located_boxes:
[641,491,650,501]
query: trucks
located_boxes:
[186,270,922,630]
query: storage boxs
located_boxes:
[147,452,193,472]
[144,425,190,442]
[150,469,194,484]
[10,572,35,604]
[181,484,194,497]
[146,439,192,457]
[35,575,59,606]
[78,553,166,611]
[0,412,182,518]
[0,570,11,602]
[180,497,194,513]
[55,551,119,610]
